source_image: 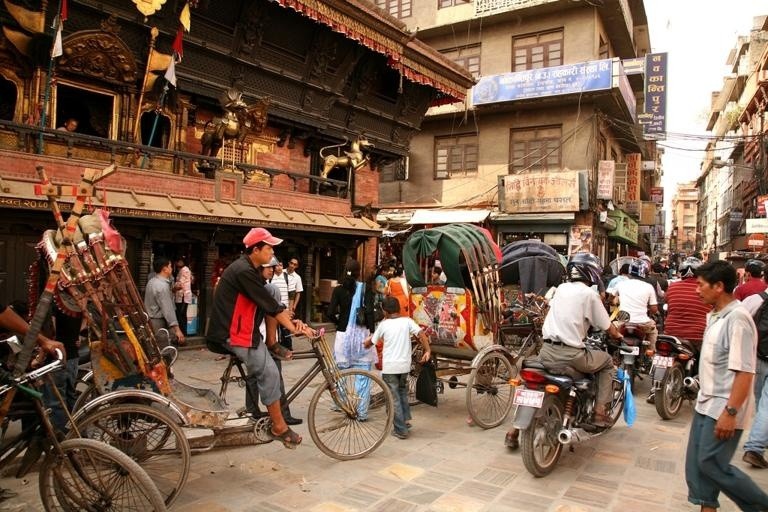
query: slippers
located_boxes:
[267,343,293,361]
[266,427,302,445]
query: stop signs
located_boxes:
[744,233,766,251]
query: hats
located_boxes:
[243,228,284,249]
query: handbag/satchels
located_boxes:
[356,304,384,324]
[417,363,438,407]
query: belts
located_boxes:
[544,339,569,346]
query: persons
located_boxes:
[268,259,304,351]
[601,252,768,469]
[505,251,625,447]
[246,253,303,421]
[1,267,84,502]
[143,254,195,347]
[56,118,77,132]
[684,260,767,512]
[328,256,447,439]
[206,228,318,445]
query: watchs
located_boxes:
[725,406,738,415]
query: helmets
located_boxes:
[567,252,765,286]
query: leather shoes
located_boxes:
[505,432,519,448]
[592,413,614,428]
[287,416,303,424]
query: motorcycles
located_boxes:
[648,334,701,420]
[619,323,649,394]
[506,310,631,479]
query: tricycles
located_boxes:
[490,282,567,377]
[0,266,546,512]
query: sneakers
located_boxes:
[647,388,657,402]
[743,452,768,469]
